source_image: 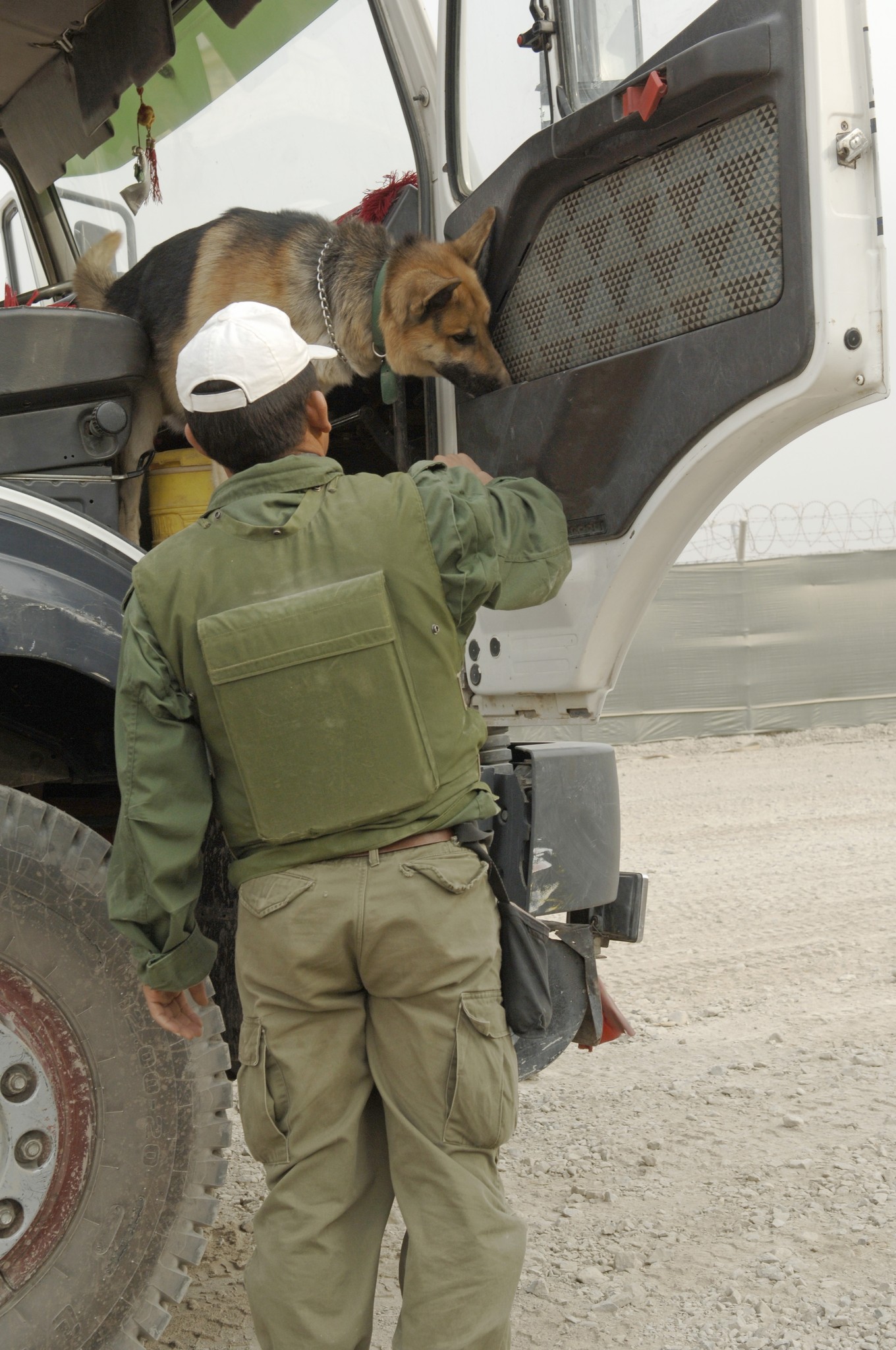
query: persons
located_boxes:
[101,299,573,1350]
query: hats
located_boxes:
[174,300,339,414]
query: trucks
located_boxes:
[0,0,892,1349]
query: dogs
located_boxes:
[76,207,512,549]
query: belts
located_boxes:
[338,826,454,858]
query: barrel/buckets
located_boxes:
[145,448,214,550]
